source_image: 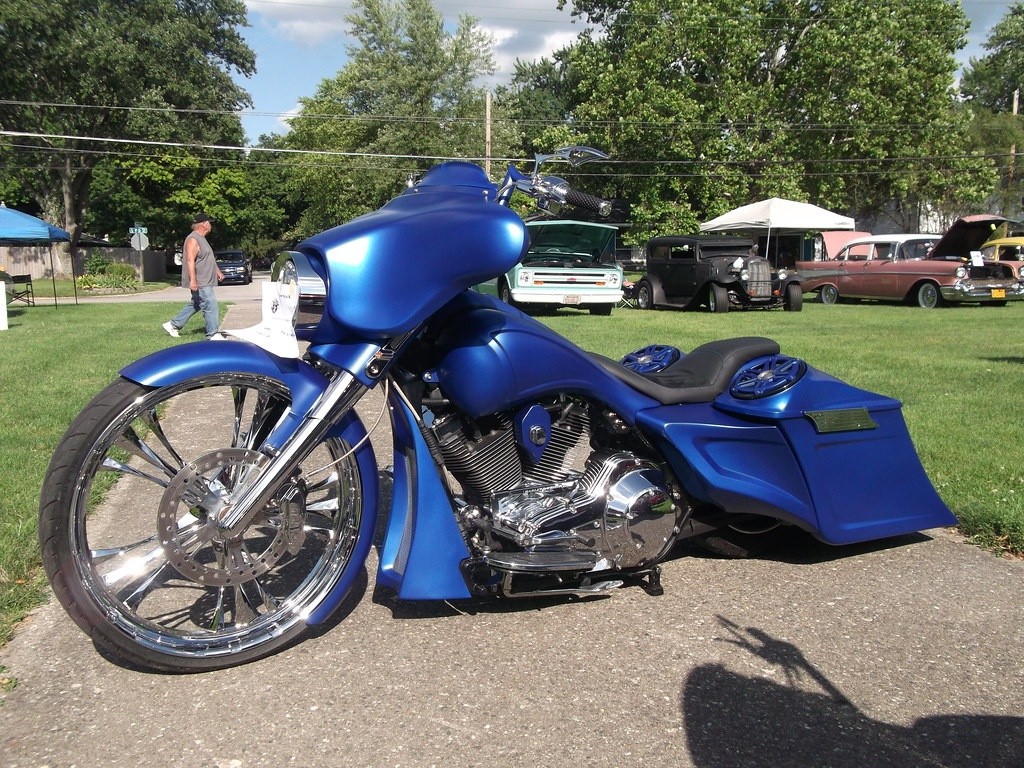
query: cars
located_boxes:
[977,236,1024,263]
[215,250,254,285]
[794,214,1024,309]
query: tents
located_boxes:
[0,206,77,309]
[699,198,855,259]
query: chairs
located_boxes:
[614,274,643,309]
[878,250,894,258]
[9,274,35,307]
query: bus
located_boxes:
[464,217,625,319]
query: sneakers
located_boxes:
[209,333,229,340]
[163,321,181,338]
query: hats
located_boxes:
[194,214,215,222]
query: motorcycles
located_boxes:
[38,143,961,673]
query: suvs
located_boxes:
[634,233,803,315]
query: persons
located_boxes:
[163,214,226,340]
[618,262,636,296]
